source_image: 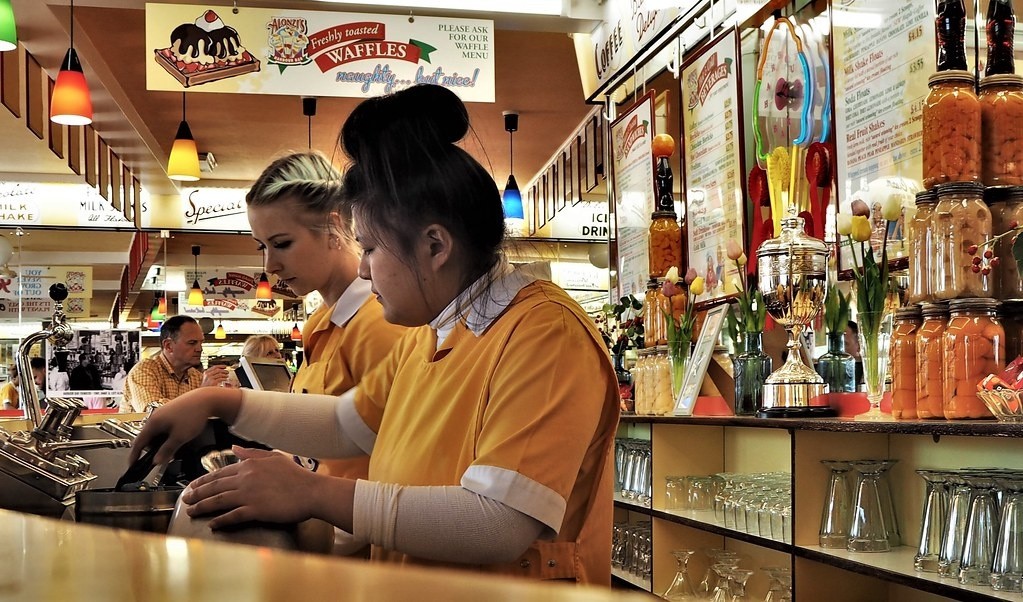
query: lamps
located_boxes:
[50,0,93,125]
[188,246,204,305]
[167,92,201,181]
[215,319,226,339]
[255,249,271,301]
[503,114,524,220]
[0,0,17,52]
[148,268,167,329]
[291,311,301,341]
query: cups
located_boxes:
[610,520,652,586]
[612,437,652,508]
[821,458,903,554]
[663,475,715,511]
[914,467,1023,593]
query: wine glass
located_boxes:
[713,471,791,545]
[658,547,793,602]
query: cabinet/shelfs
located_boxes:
[610,414,1023,601]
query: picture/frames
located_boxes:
[528,106,607,236]
[673,303,734,415]
[0,37,141,232]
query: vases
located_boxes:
[856,312,893,406]
[817,331,855,393]
[666,341,692,402]
[733,332,772,416]
[611,354,630,386]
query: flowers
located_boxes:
[659,265,705,399]
[824,259,851,390]
[727,240,766,411]
[596,294,644,356]
[837,193,901,395]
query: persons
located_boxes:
[49,353,103,390]
[127,84,621,590]
[124,315,235,427]
[30,357,46,410]
[239,335,282,368]
[0,364,19,410]
[245,149,416,554]
